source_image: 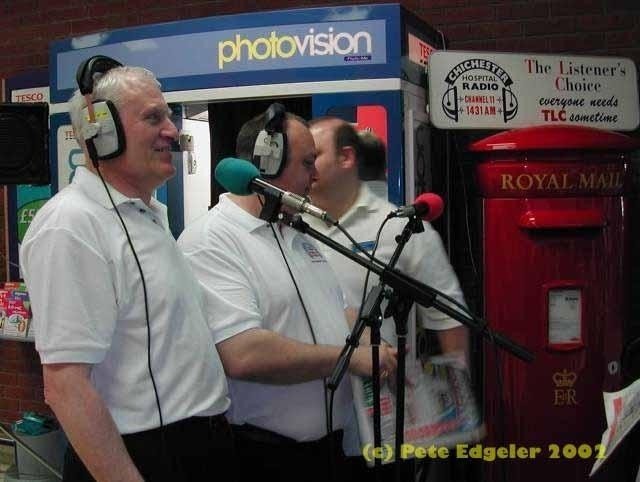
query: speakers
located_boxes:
[0,101,51,185]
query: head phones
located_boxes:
[76,55,125,160]
[251,102,287,180]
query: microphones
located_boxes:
[388,192,445,222]
[214,157,338,225]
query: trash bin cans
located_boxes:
[13,429,65,481]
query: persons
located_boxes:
[176,103,471,482]
[19,66,239,482]
